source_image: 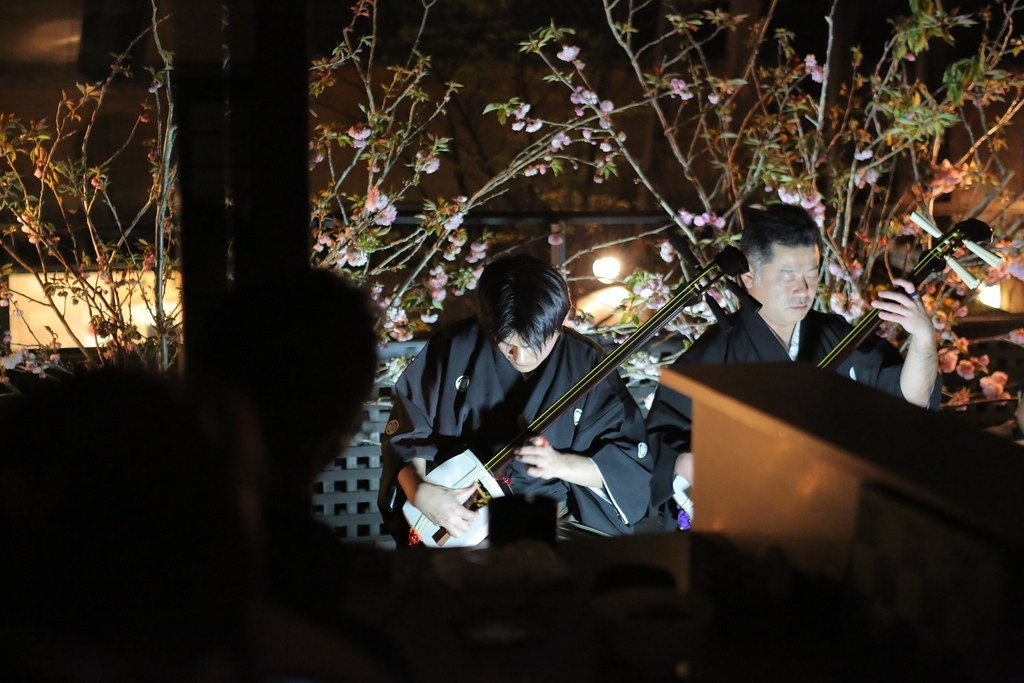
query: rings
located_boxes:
[537,433,546,447]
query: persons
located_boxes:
[0,265,381,683]
[644,203,937,532]
[380,254,654,548]
